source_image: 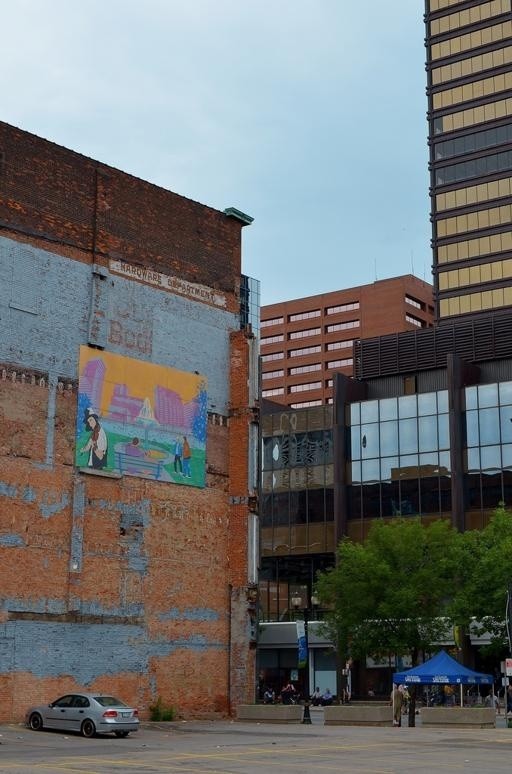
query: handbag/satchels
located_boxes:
[93,448,107,467]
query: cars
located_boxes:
[25,692,141,740]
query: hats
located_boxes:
[82,407,98,423]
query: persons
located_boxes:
[256,673,511,727]
[124,436,144,474]
[181,434,193,479]
[80,407,109,472]
[172,436,184,474]
